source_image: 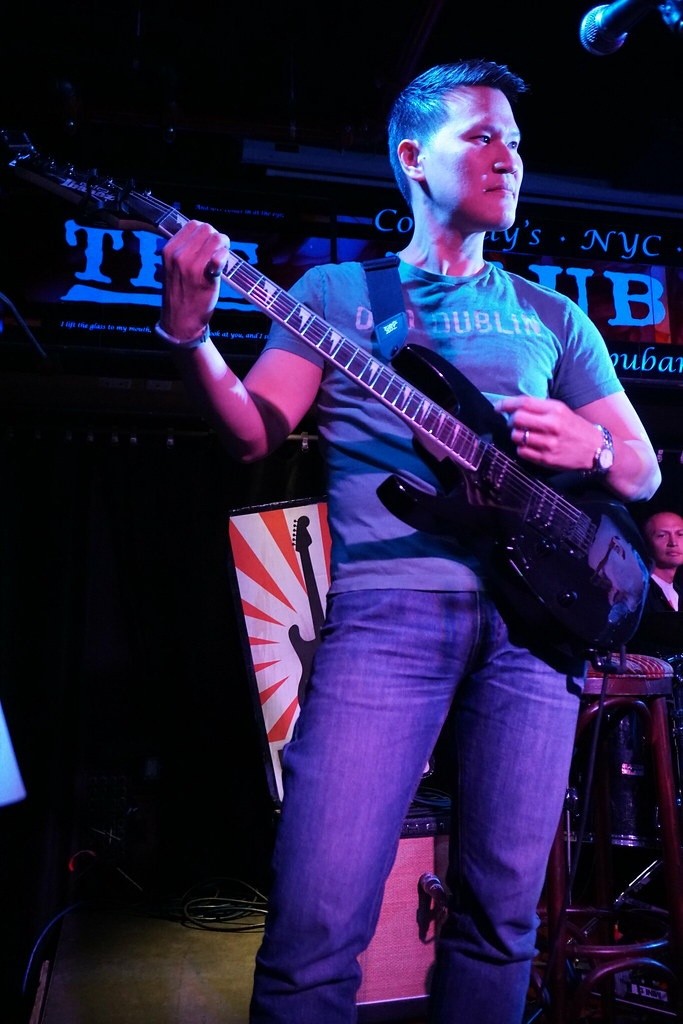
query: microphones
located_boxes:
[420,871,446,902]
[579,0,652,57]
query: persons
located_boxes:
[152,62,661,1024]
[629,512,683,662]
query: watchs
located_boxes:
[579,425,614,476]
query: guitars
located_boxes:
[8,133,656,657]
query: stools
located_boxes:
[546,653,683,1024]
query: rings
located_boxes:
[524,430,530,447]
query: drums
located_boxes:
[562,732,585,843]
[606,651,682,852]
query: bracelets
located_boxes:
[155,321,210,350]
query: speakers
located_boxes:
[354,796,452,1007]
